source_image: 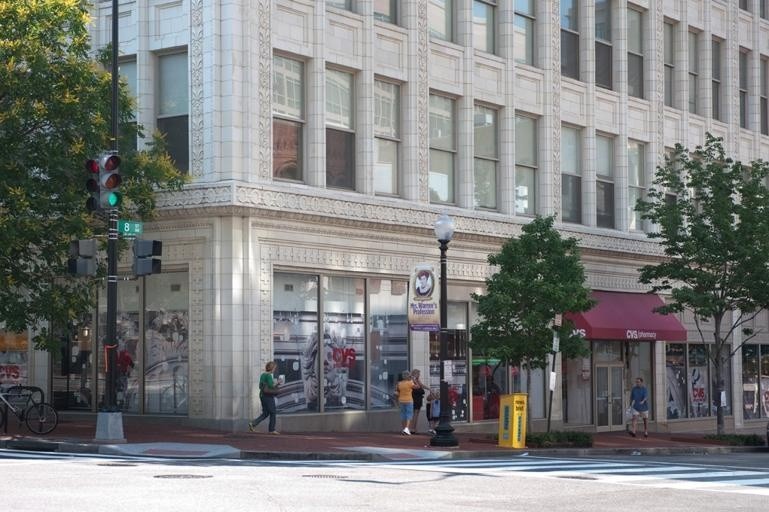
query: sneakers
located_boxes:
[644,433,648,438]
[410,429,418,434]
[401,428,411,436]
[270,430,280,435]
[249,421,255,432]
[630,430,635,438]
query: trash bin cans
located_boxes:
[498,392,528,448]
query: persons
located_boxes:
[412,369,432,433]
[303,326,349,399]
[628,378,650,437]
[98,347,135,408]
[396,370,421,436]
[415,271,431,295]
[427,390,440,435]
[455,365,500,419]
[248,359,283,436]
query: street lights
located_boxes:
[429,210,464,444]
[743,348,752,377]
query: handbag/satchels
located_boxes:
[259,379,283,395]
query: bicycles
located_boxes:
[158,366,187,413]
[0,380,59,434]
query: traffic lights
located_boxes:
[135,240,162,273]
[103,156,124,210]
[85,158,103,210]
[66,240,89,273]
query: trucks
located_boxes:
[741,381,765,417]
